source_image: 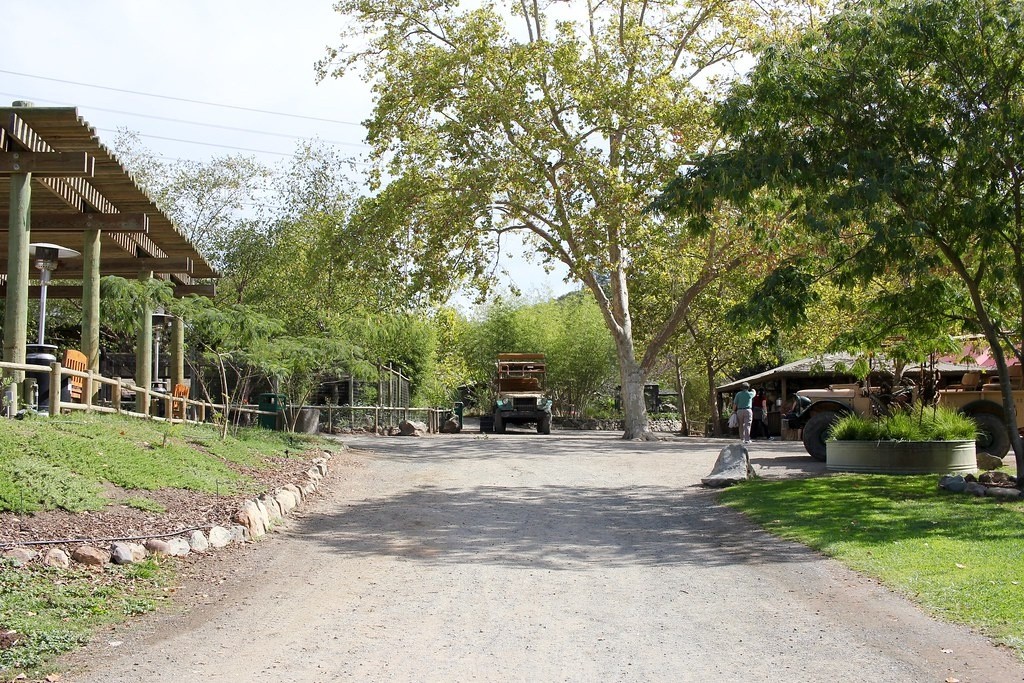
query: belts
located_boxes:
[737,408,752,410]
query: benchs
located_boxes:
[944,373,979,392]
[981,365,1024,391]
[61,349,87,399]
[172,383,189,419]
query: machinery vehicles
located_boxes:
[780,335,1024,460]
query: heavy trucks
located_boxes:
[480,353,552,435]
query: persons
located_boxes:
[789,397,804,440]
[732,382,756,443]
[752,387,774,441]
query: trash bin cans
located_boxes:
[454,402,464,429]
[257,393,286,431]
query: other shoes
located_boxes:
[745,440,752,445]
[741,439,745,443]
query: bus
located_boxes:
[99,352,194,416]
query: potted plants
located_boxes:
[823,400,988,474]
